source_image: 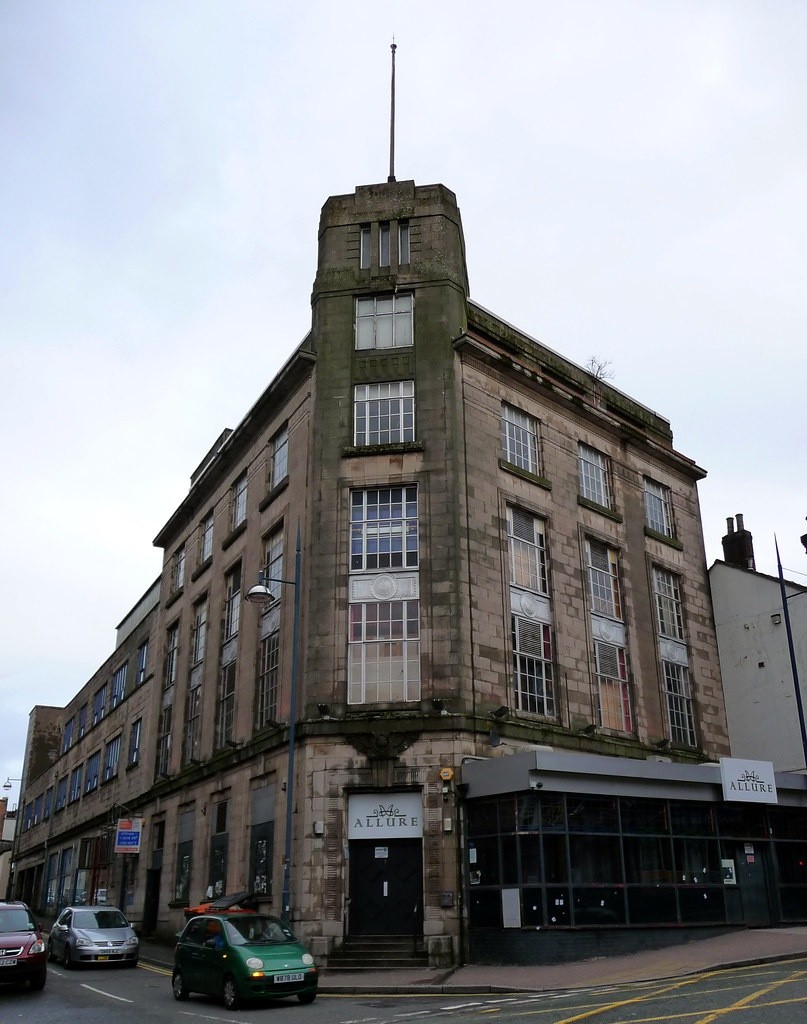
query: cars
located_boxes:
[0,900,47,992]
[172,912,319,1011]
[47,905,139,970]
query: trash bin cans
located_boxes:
[184,892,257,942]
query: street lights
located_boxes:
[245,569,302,929]
[2,777,28,898]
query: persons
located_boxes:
[212,923,236,950]
